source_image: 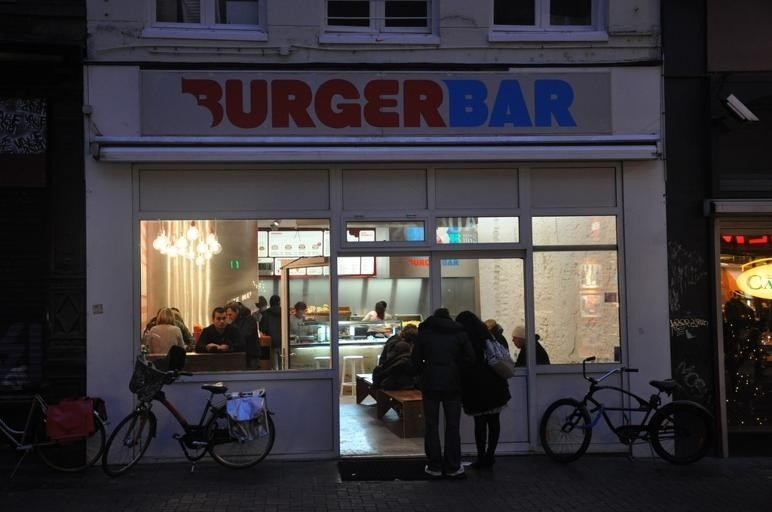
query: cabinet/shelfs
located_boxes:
[290,307,352,339]
[349,314,424,339]
[288,320,401,396]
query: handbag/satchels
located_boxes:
[482,337,514,379]
[128,356,168,401]
[44,397,108,443]
[226,388,270,442]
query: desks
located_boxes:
[145,351,247,371]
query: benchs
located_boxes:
[355,373,426,440]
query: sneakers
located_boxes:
[445,464,464,476]
[424,465,441,475]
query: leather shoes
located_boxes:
[469,457,496,470]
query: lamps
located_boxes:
[152,220,223,267]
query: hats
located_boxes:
[511,326,525,339]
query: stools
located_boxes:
[314,356,331,370]
[340,355,364,399]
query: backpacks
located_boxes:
[376,342,416,387]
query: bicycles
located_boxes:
[101,346,277,479]
[0,367,106,485]
[538,355,721,468]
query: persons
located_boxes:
[290,302,306,335]
[361,300,550,477]
[143,295,281,371]
